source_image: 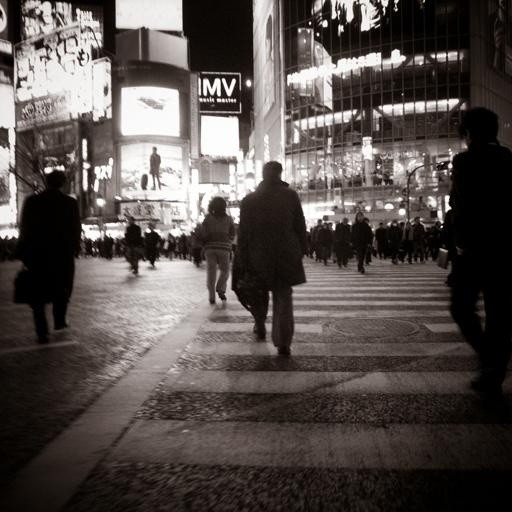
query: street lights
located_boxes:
[406,160,450,220]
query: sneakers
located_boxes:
[278,346,290,355]
[210,291,226,303]
[253,325,265,338]
[39,323,68,343]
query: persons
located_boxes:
[305,212,454,274]
[448,105,511,395]
[150,147,162,190]
[201,197,236,304]
[238,163,306,354]
[80,215,240,273]
[18,172,81,339]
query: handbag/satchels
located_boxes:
[13,268,29,304]
